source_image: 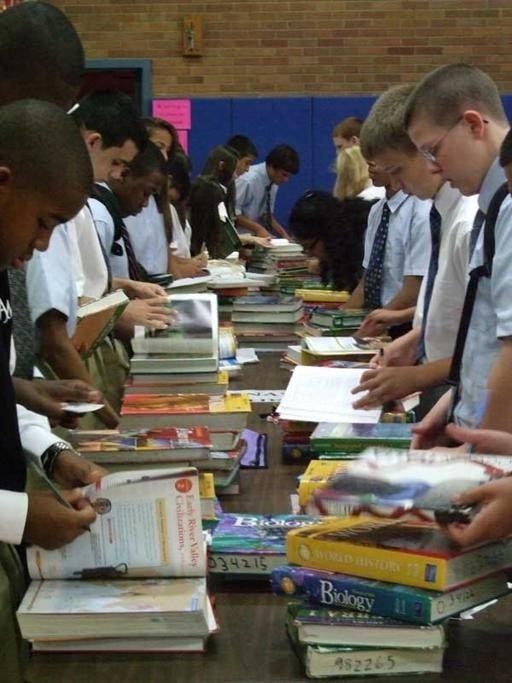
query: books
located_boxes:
[192,235,512,683]
[16,466,218,653]
[131,292,220,373]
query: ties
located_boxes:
[121,223,139,281]
[416,203,441,361]
[266,184,271,232]
[364,203,390,311]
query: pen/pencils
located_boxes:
[43,361,59,380]
[200,241,206,253]
[30,461,91,533]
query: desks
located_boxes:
[21,234,512,683]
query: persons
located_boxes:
[346,158,435,340]
[332,141,372,197]
[191,145,273,262]
[404,60,512,451]
[228,134,258,179]
[235,143,301,240]
[348,80,479,408]
[441,127,512,546]
[2,1,88,105]
[1,84,210,548]
[331,114,365,151]
[289,188,376,292]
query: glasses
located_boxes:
[420,116,488,162]
[301,238,319,254]
